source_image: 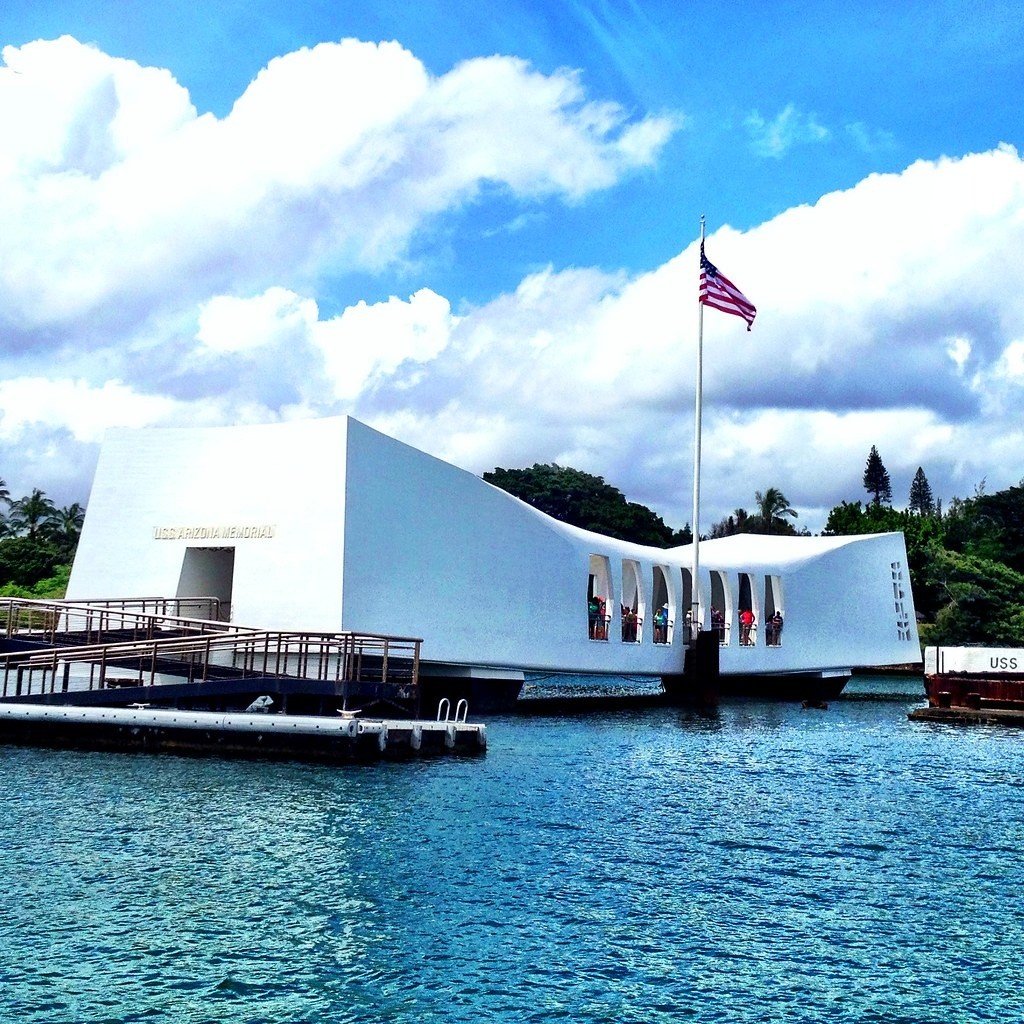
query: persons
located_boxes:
[586,596,785,646]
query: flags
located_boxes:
[696,248,760,332]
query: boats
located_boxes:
[923,643,1024,708]
